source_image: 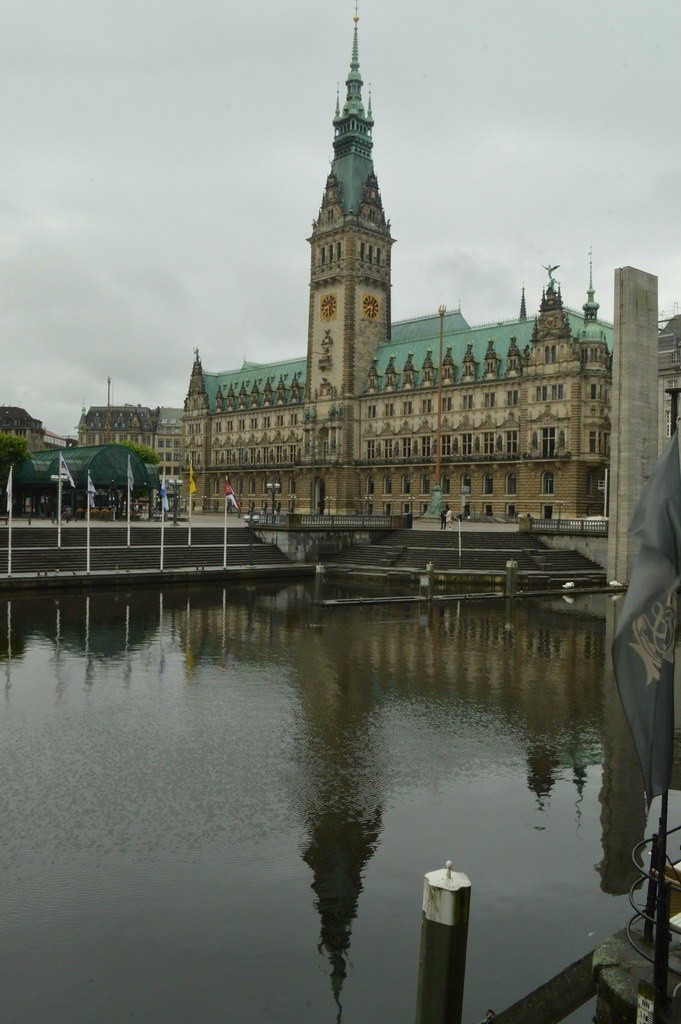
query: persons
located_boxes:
[65,505,72,522]
[440,506,453,530]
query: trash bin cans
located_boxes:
[244,515,251,527]
[402,513,412,529]
[252,515,260,527]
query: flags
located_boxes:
[161,478,170,511]
[60,453,76,488]
[225,476,241,511]
[609,426,681,814]
[127,455,134,491]
[6,466,12,511]
[190,460,196,494]
[88,469,96,508]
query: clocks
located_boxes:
[360,290,381,321]
[319,291,338,322]
